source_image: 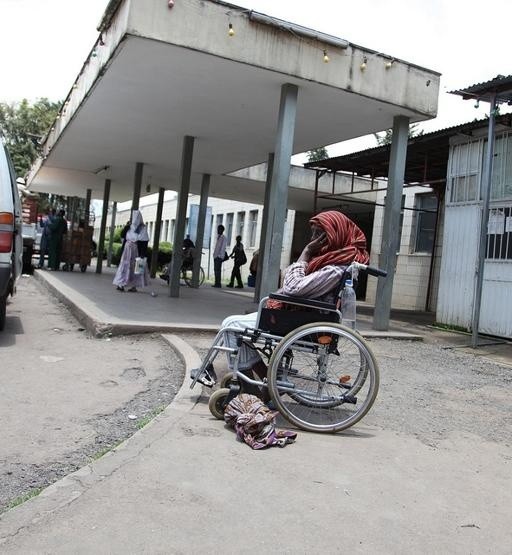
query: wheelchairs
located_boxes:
[187,261,392,436]
[161,247,207,289]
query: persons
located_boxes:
[113,209,243,291]
[220,210,370,404]
[35,208,67,270]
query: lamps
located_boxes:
[94,165,110,175]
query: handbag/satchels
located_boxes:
[241,250,247,265]
[224,252,229,261]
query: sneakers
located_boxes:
[116,286,137,292]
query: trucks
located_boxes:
[0,133,55,331]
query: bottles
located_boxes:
[341,279,356,329]
[139,257,144,271]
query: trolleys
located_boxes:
[56,209,96,273]
